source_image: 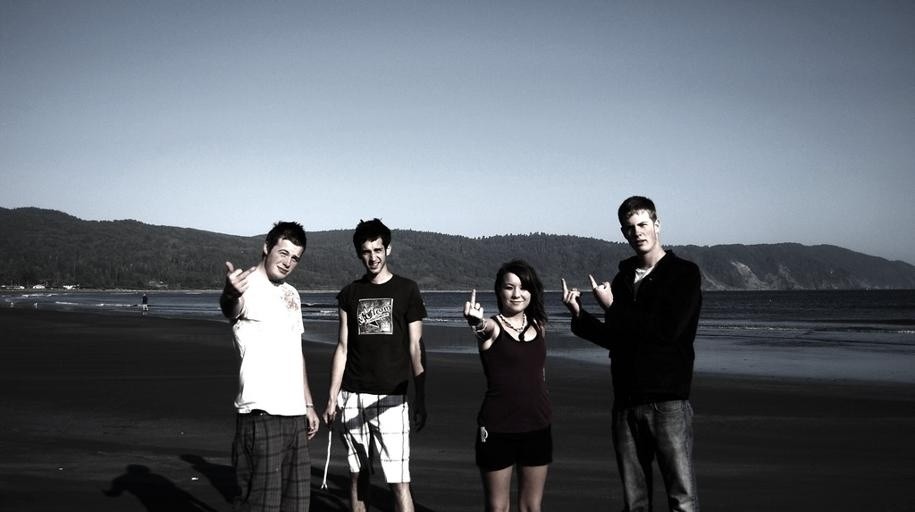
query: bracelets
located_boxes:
[305,404,315,408]
[471,321,487,333]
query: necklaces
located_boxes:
[497,312,525,331]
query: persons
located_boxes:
[321,217,426,512]
[463,256,553,512]
[220,222,322,512]
[562,195,703,512]
[142,291,149,312]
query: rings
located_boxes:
[474,307,479,311]
